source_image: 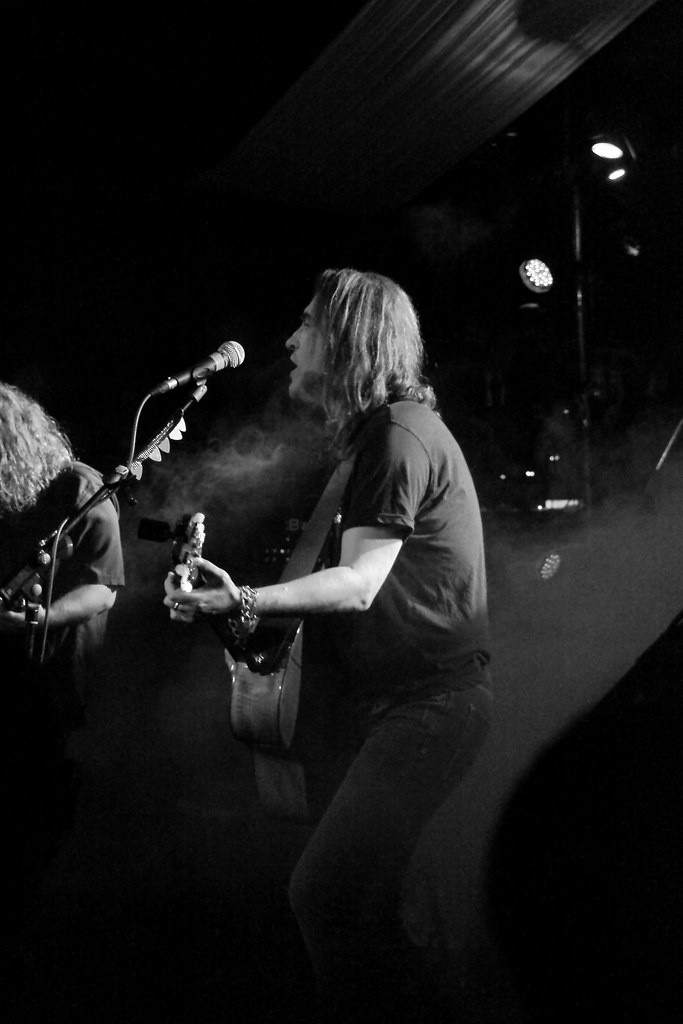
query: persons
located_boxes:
[164,267,496,1024]
[0,383,126,904]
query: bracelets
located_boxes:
[238,586,257,625]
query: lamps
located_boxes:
[590,131,636,187]
[516,246,577,293]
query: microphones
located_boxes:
[151,338,247,394]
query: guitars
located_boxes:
[0,528,75,617]
[169,509,339,827]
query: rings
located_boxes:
[174,603,178,609]
